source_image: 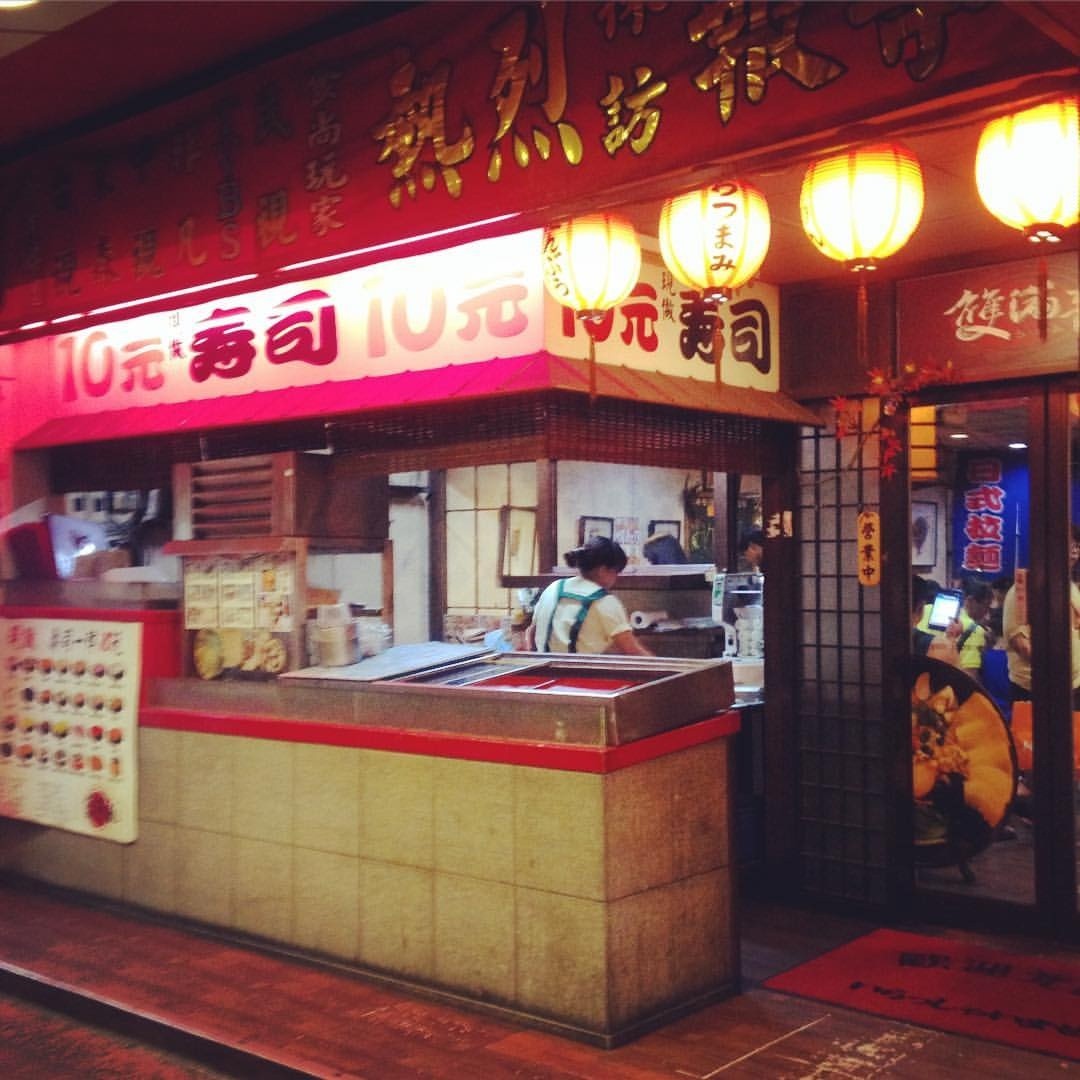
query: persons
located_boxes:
[737,523,762,602]
[917,575,994,678]
[923,580,941,604]
[527,536,652,657]
[1003,523,1080,801]
[912,574,1015,840]
[984,577,1012,637]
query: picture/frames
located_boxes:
[650,520,680,540]
[912,501,938,567]
[579,516,613,545]
[499,506,539,575]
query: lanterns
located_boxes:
[658,177,771,391]
[975,95,1080,343]
[800,141,924,368]
[541,212,643,411]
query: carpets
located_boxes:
[761,927,1080,1060]
